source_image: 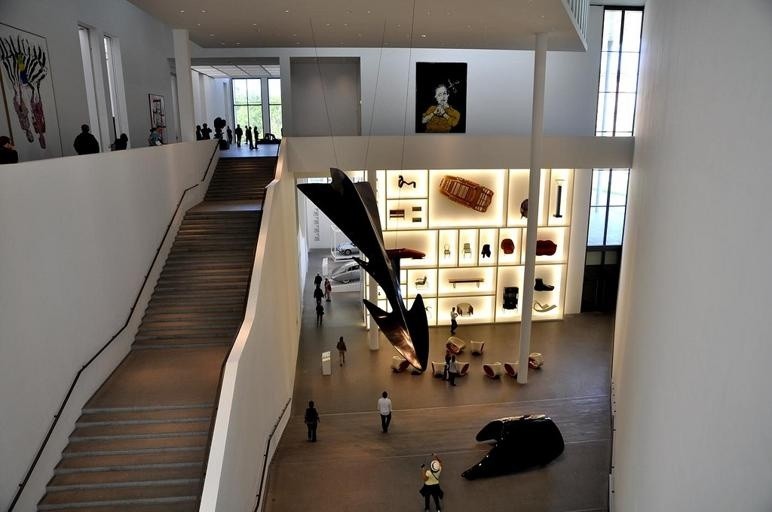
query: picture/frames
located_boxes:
[0,22,64,163]
[149,93,168,144]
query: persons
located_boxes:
[110,133,128,151]
[449,355,457,386]
[196,123,259,150]
[0,136,19,164]
[445,350,451,370]
[304,401,321,442]
[422,84,460,133]
[336,336,347,365]
[148,126,163,146]
[451,307,458,335]
[376,391,393,433]
[74,124,100,155]
[421,452,442,512]
[314,271,332,328]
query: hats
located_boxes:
[430,460,441,472]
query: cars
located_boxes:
[331,259,360,283]
[337,241,359,255]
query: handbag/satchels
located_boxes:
[438,487,444,499]
